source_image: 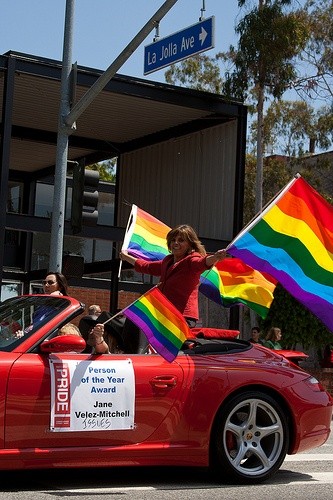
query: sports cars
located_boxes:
[0,293,333,485]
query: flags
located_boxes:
[121,204,279,320]
[122,285,194,365]
[225,174,333,334]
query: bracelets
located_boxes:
[95,339,104,345]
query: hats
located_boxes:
[81,311,126,352]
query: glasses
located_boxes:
[42,280,58,285]
[251,332,257,333]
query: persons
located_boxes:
[248,326,263,345]
[33,272,79,331]
[0,305,125,354]
[261,327,282,350]
[122,224,226,355]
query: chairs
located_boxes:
[103,315,147,354]
[79,315,98,346]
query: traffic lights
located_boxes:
[69,164,99,227]
